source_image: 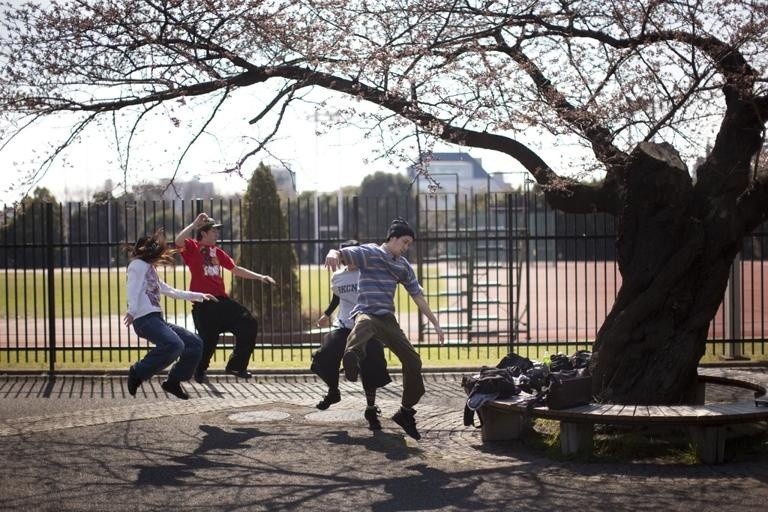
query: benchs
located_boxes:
[464,360,767,466]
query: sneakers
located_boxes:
[315,390,340,410]
[128,366,142,395]
[343,349,358,382]
[391,407,421,441]
[194,369,204,383]
[161,379,188,400]
[364,405,381,420]
[225,365,251,378]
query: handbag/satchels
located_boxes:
[549,354,573,372]
[476,366,514,393]
[545,373,592,410]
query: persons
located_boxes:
[175,213,275,383]
[311,239,392,424]
[119,228,219,399]
[324,216,444,441]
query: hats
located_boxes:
[198,217,223,230]
[386,217,414,241]
[133,237,162,256]
